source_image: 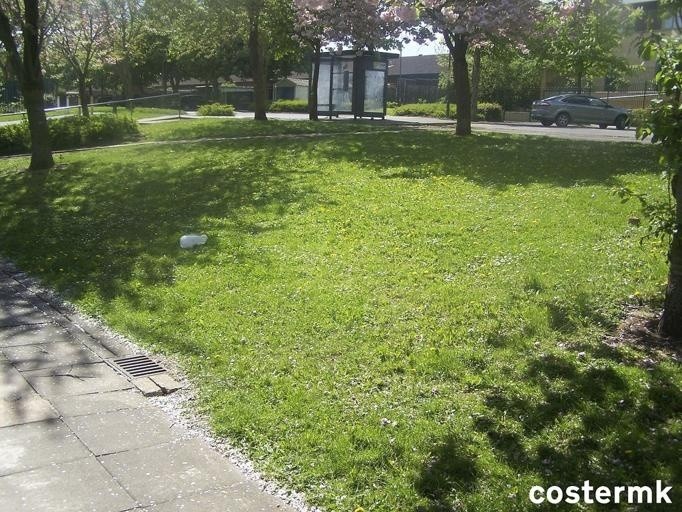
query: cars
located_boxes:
[528,92,635,129]
